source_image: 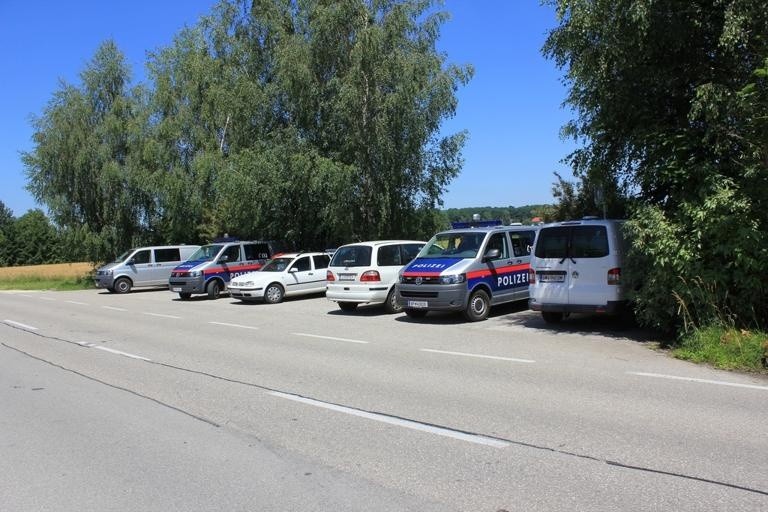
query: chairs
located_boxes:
[458,236,477,250]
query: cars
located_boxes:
[227,251,334,304]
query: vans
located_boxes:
[325,216,624,332]
[95,240,275,299]
